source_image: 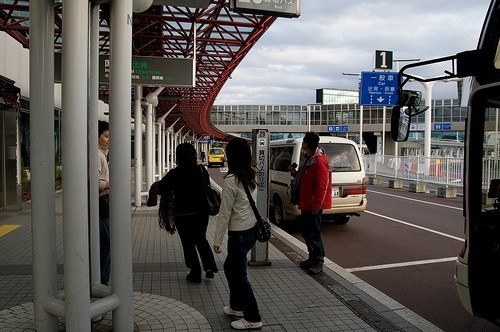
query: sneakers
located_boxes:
[223,305,244,317]
[230,318,262,330]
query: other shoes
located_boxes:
[186,272,202,283]
[205,271,214,278]
[300,259,314,270]
[308,265,324,275]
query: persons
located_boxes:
[97,122,110,286]
[214,137,263,330]
[288,132,332,274]
[147,143,218,282]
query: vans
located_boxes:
[268,136,368,230]
[207,147,226,168]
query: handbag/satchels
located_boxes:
[289,179,301,206]
[199,164,221,215]
[256,219,271,242]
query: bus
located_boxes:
[391,0,500,327]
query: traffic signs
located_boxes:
[359,71,404,107]
